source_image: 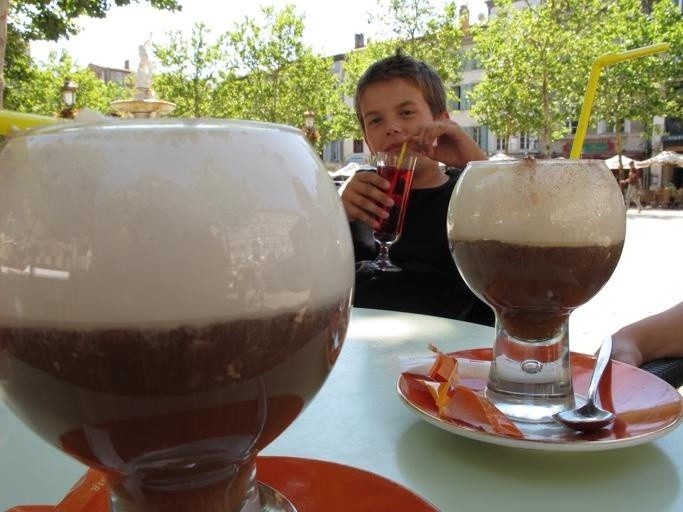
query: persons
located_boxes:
[594,297,683,366]
[619,161,642,213]
[331,56,494,330]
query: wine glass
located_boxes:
[360,152,418,275]
[447,160,627,423]
[4,120,353,510]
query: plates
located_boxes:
[53,454,440,511]
[395,347,682,452]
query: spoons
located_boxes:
[552,335,624,431]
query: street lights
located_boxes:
[57,74,80,118]
[301,106,319,149]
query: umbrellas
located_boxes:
[603,154,642,169]
[636,148,683,180]
